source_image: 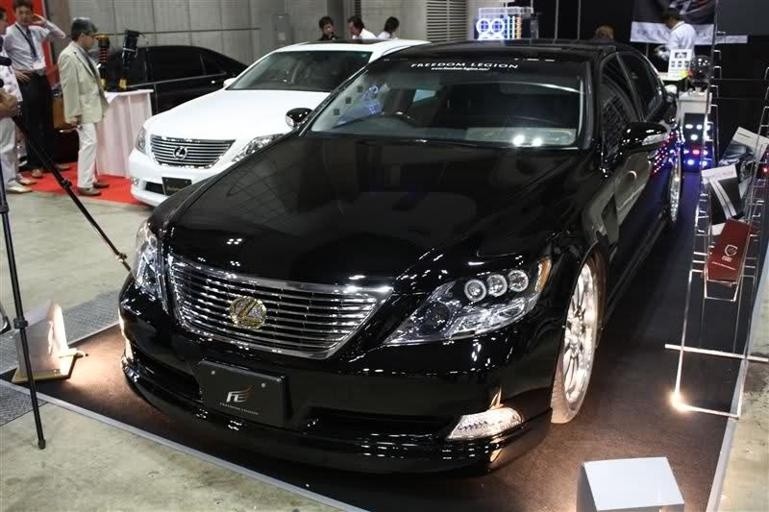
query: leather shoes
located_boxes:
[19,176,36,184]
[6,181,31,193]
[56,163,71,170]
[78,186,101,195]
[31,169,43,178]
[95,180,110,188]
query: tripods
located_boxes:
[0,79,131,449]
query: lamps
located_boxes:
[474,18,506,40]
[570,454,686,512]
[11,298,75,375]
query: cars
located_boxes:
[654,45,670,60]
[16,44,249,172]
[115,37,686,483]
[126,38,434,210]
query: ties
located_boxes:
[26,27,37,61]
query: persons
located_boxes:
[655,8,698,61]
[0,88,17,118]
[318,16,339,40]
[376,15,399,38]
[593,25,615,41]
[0,7,36,194]
[2,0,71,178]
[347,15,376,39]
[57,16,111,196]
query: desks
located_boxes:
[90,88,158,180]
[660,71,688,98]
[678,90,714,142]
